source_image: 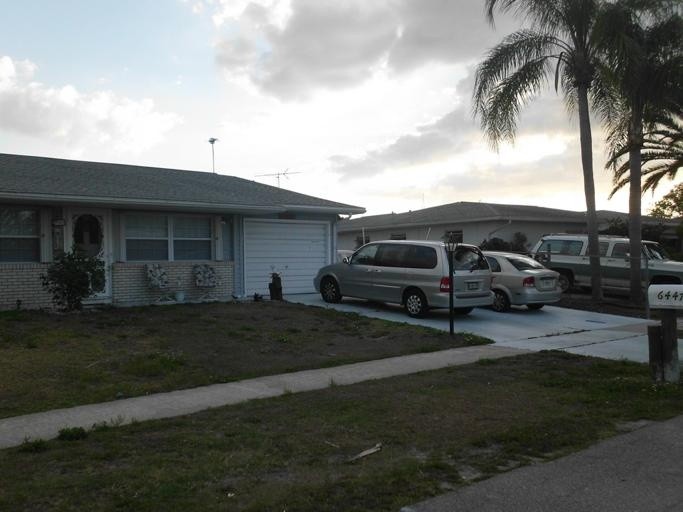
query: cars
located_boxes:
[481,250,560,312]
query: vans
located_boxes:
[533,233,682,301]
[312,239,495,320]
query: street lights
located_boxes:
[443,232,458,337]
